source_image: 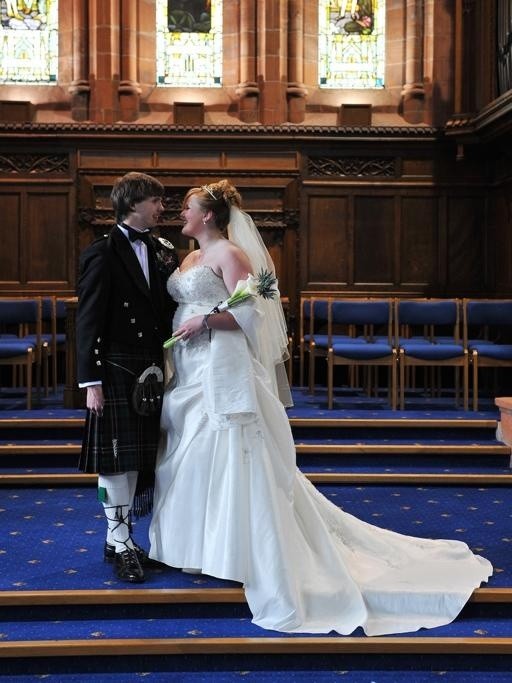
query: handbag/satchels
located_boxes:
[202,314,210,331]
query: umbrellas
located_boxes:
[104,540,146,564]
[113,548,146,583]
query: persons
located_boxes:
[166,179,493,638]
[73,172,180,582]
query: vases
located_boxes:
[158,266,284,351]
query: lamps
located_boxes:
[0,278,75,410]
[298,294,511,411]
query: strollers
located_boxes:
[120,223,156,246]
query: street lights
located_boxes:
[133,361,165,416]
